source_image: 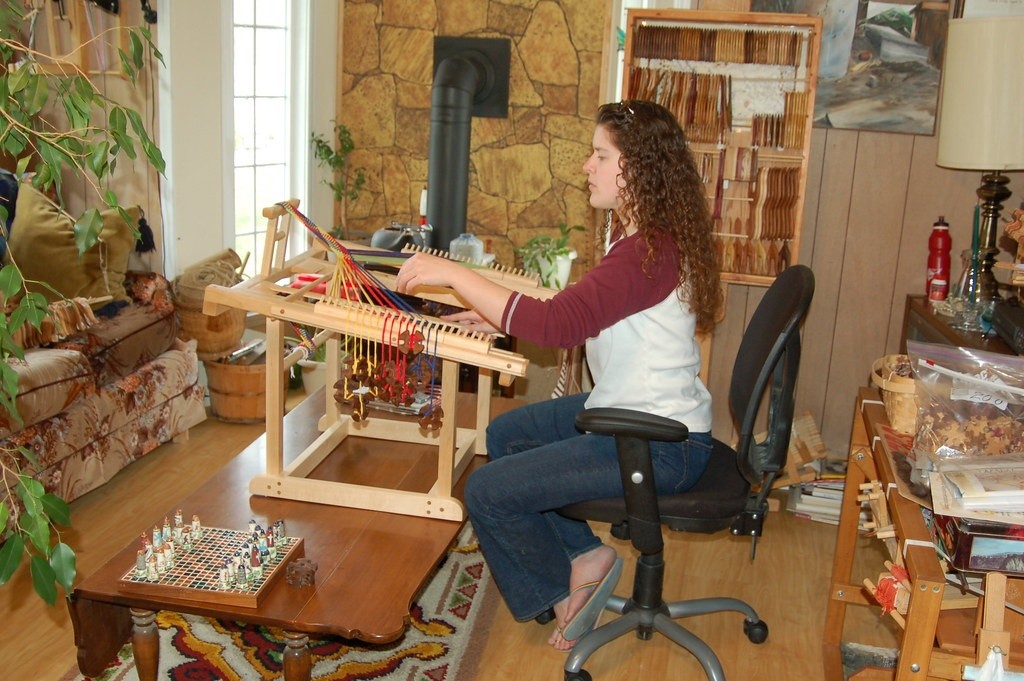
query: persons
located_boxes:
[397,100,723,651]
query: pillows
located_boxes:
[102,203,145,304]
[4,181,109,303]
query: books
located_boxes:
[786,474,871,533]
[929,462,1024,526]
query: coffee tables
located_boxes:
[61,380,529,681]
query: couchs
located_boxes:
[0,271,209,539]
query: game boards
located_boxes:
[116,522,305,609]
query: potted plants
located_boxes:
[514,220,589,290]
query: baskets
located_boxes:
[871,353,950,436]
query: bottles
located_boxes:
[982,252,1002,323]
[929,272,947,303]
[926,216,952,299]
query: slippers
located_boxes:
[553,602,608,652]
[561,556,625,641]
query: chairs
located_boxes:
[563,264,817,681]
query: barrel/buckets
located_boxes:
[203,351,269,424]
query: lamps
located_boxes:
[935,15,1024,304]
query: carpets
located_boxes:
[63,521,501,681]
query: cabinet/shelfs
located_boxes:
[822,385,1024,681]
[898,293,1018,364]
